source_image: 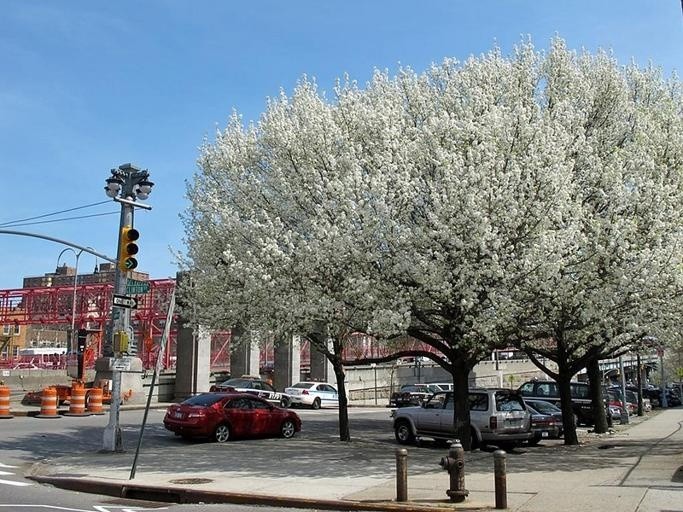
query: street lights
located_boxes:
[103,163,154,452]
[57,246,98,354]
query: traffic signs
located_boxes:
[113,294,138,309]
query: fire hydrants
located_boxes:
[439,439,469,502]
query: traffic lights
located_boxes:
[118,225,140,272]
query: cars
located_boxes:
[391,377,683,451]
[163,375,349,443]
